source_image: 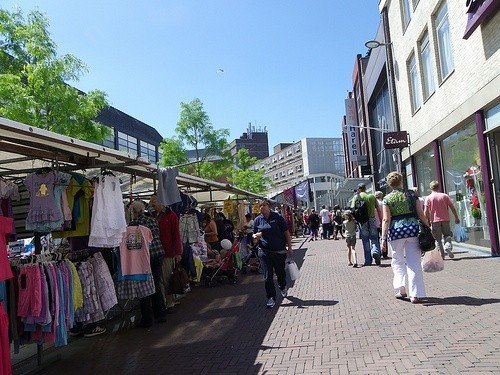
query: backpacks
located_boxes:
[353,195,369,223]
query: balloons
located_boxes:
[221,239,232,249]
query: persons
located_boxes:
[252,200,292,308]
[351,183,383,266]
[202,213,218,250]
[206,249,228,269]
[375,191,388,259]
[218,212,237,275]
[245,213,254,243]
[343,210,357,267]
[293,205,348,241]
[413,188,424,210]
[68,234,106,336]
[194,243,219,286]
[247,239,257,253]
[150,193,183,312]
[381,172,436,303]
[424,181,460,259]
[131,201,166,327]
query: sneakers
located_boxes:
[266,298,274,305]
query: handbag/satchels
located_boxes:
[288,257,300,281]
[334,215,342,225]
[418,221,435,251]
[170,262,188,289]
[453,224,470,242]
[421,246,444,272]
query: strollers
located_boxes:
[240,232,263,274]
[200,232,245,288]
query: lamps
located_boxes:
[365,41,393,48]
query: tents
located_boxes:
[0,117,276,366]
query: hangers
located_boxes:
[127,218,140,226]
[23,252,64,266]
[36,159,52,174]
[92,162,123,184]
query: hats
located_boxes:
[344,210,352,215]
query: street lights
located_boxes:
[344,124,402,173]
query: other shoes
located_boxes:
[411,297,422,303]
[84,326,107,337]
[395,293,407,297]
[348,263,353,266]
[281,286,286,298]
[364,263,371,266]
[373,253,381,265]
[353,264,358,267]
[446,248,454,258]
[69,327,85,335]
[328,238,331,239]
[383,255,388,259]
[136,320,151,327]
[334,238,339,240]
